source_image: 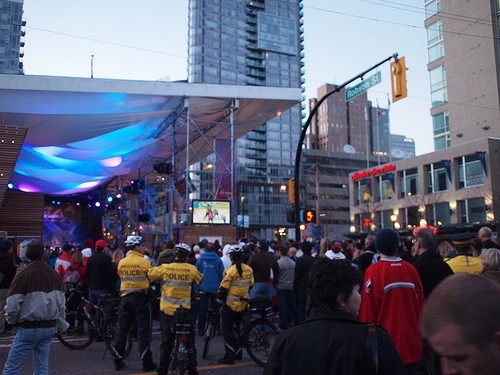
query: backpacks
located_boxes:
[62,265,84,294]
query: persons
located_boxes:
[204,206,226,223]
[0,227,500,375]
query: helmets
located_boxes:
[227,245,243,255]
[172,243,192,254]
[124,235,144,249]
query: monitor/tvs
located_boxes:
[191,199,231,225]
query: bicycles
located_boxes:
[196,291,283,365]
[57,289,133,360]
[167,321,193,375]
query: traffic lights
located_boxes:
[303,210,317,224]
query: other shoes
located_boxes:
[234,354,242,359]
[219,358,234,364]
[95,334,104,342]
[143,362,157,371]
[77,330,84,336]
[115,361,125,370]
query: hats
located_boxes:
[374,228,399,250]
[451,233,476,245]
[62,244,74,252]
[95,240,109,248]
[25,242,44,255]
